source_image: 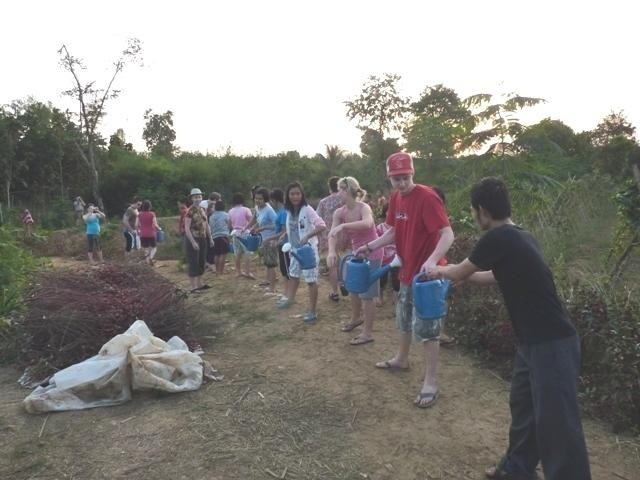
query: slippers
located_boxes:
[190,284,213,294]
[440,336,460,348]
[340,285,349,296]
[278,295,295,308]
[349,334,375,346]
[414,385,440,408]
[302,310,320,322]
[374,356,411,372]
[339,319,366,332]
[483,462,538,480]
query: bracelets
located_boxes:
[366,242,371,253]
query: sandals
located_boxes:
[329,292,342,301]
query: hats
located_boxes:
[385,152,416,178]
[187,188,205,197]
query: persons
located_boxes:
[241,187,291,301]
[178,187,254,293]
[354,153,452,408]
[315,177,375,300]
[377,187,453,347]
[122,197,143,263]
[81,202,105,265]
[134,200,162,265]
[427,176,592,480]
[22,209,34,236]
[276,180,327,321]
[327,176,382,344]
[73,195,86,227]
